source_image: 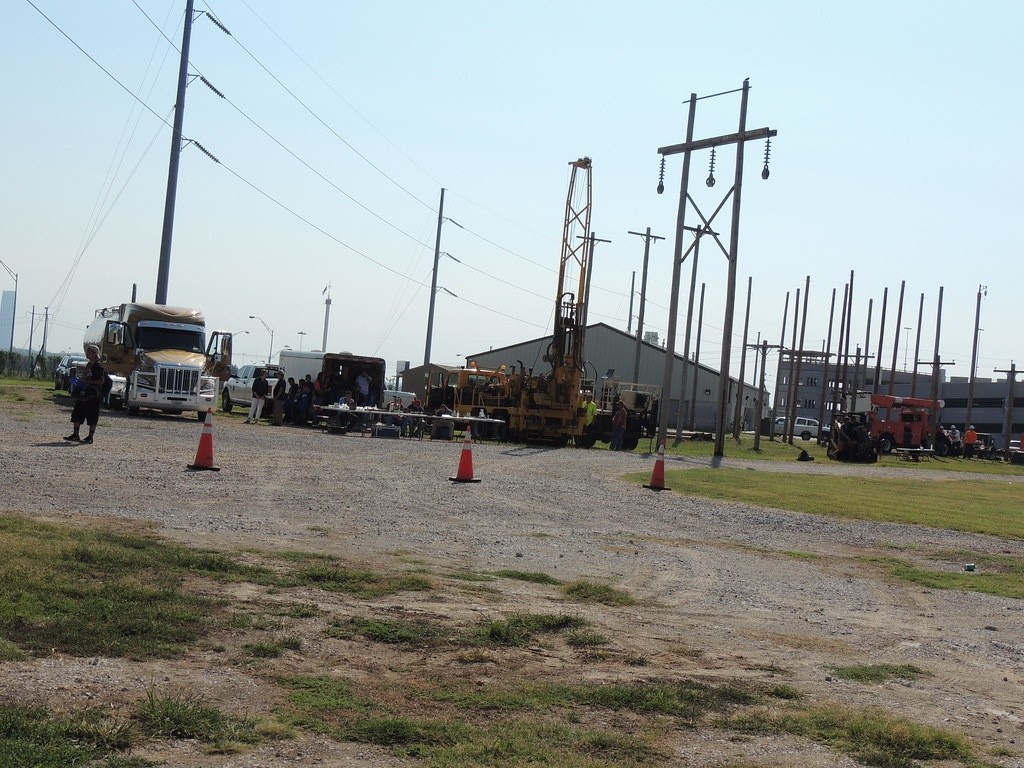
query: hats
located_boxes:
[276,370,285,375]
[414,400,420,405]
[940,426,944,429]
[969,425,974,429]
[951,425,956,429]
[88,344,100,359]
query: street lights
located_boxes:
[904,327,912,372]
[298,331,306,351]
[269,349,292,364]
[975,329,984,376]
[250,361,265,365]
[249,316,274,364]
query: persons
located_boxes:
[964,425,977,458]
[387,397,406,436]
[244,369,268,424]
[611,401,627,451]
[430,405,455,440]
[577,395,597,448]
[355,370,372,405]
[841,416,860,428]
[271,372,326,426]
[339,391,355,409]
[63,345,113,445]
[938,425,960,458]
[406,399,426,437]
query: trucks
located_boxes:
[222,349,386,431]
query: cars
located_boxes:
[1009,439,1021,458]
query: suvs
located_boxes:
[55,355,89,390]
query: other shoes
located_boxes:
[430,435,435,439]
[283,421,305,427]
[347,430,352,433]
[447,437,450,440]
[244,421,250,424]
[400,433,418,438]
[251,420,257,424]
[270,423,282,426]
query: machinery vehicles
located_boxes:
[826,394,996,460]
[424,156,593,446]
[574,361,660,450]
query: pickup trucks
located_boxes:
[775,417,830,441]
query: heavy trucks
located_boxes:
[68,283,233,422]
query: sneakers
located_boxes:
[79,437,93,444]
[63,434,80,441]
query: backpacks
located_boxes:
[798,450,810,461]
[85,362,113,397]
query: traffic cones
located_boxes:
[642,439,671,490]
[186,407,221,472]
[448,425,481,483]
